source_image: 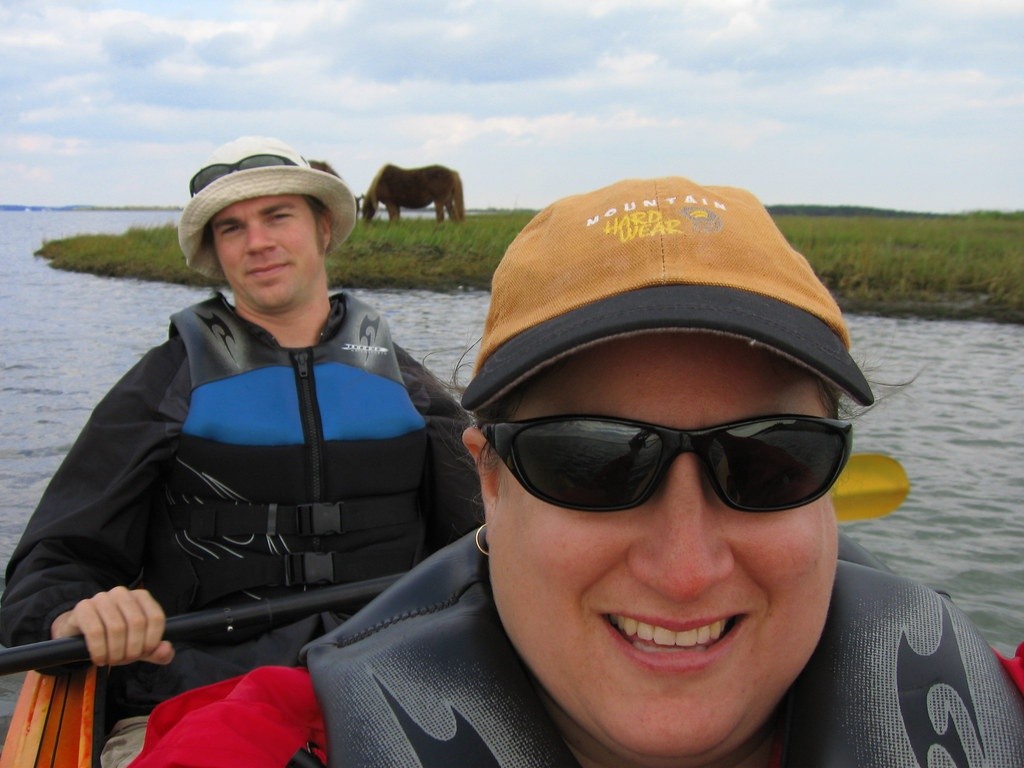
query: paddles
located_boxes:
[0,456,910,678]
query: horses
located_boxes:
[361,163,466,232]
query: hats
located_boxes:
[178,136,361,282]
[462,175,873,412]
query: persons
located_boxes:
[0,135,484,768]
[127,179,1024,768]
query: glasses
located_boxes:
[470,408,852,514]
[186,153,298,196]
